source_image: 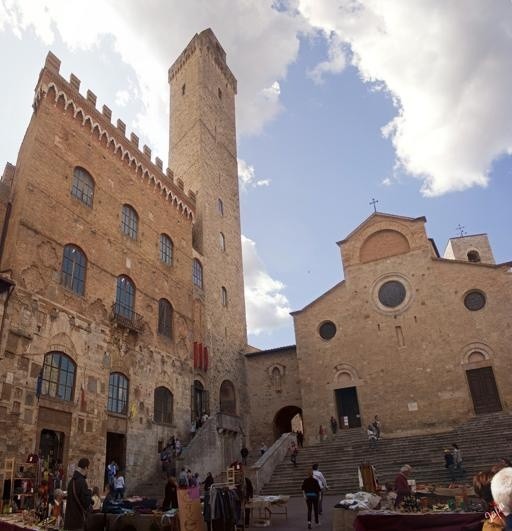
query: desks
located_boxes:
[356,511,485,531]
[245,496,289,522]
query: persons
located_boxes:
[161,435,181,471]
[319,425,323,442]
[106,459,125,500]
[472,459,512,531]
[240,445,248,465]
[163,468,214,511]
[35,447,67,521]
[368,415,381,440]
[302,464,328,529]
[395,464,413,506]
[296,430,303,448]
[330,416,337,434]
[240,471,253,528]
[192,412,208,438]
[443,443,464,483]
[64,458,94,531]
[260,442,268,454]
[288,441,298,467]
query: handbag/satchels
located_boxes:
[83,513,106,531]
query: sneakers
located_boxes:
[308,522,311,529]
